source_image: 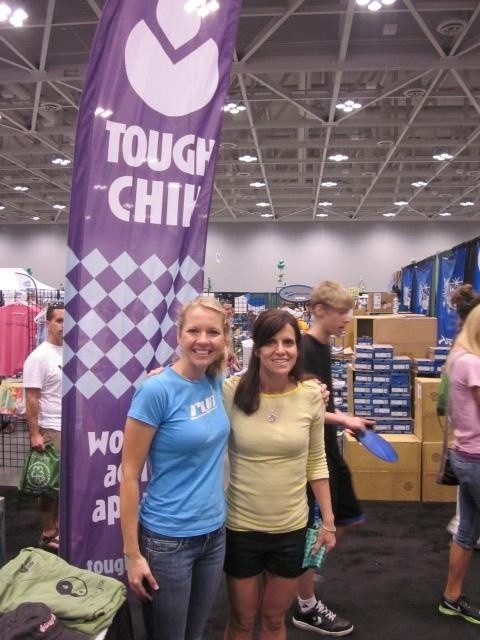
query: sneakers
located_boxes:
[437,591,480,625]
[291,599,356,638]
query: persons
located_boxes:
[437,305,480,625]
[291,282,375,636]
[146,308,337,640]
[120,295,331,640]
[221,300,236,378]
[439,284,479,547]
[23,302,63,551]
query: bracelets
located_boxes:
[321,524,337,534]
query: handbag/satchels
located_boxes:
[435,446,464,488]
[19,441,62,501]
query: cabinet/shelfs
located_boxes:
[347,315,438,358]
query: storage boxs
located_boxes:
[342,377,458,502]
[368,292,394,312]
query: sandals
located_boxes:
[38,528,60,550]
